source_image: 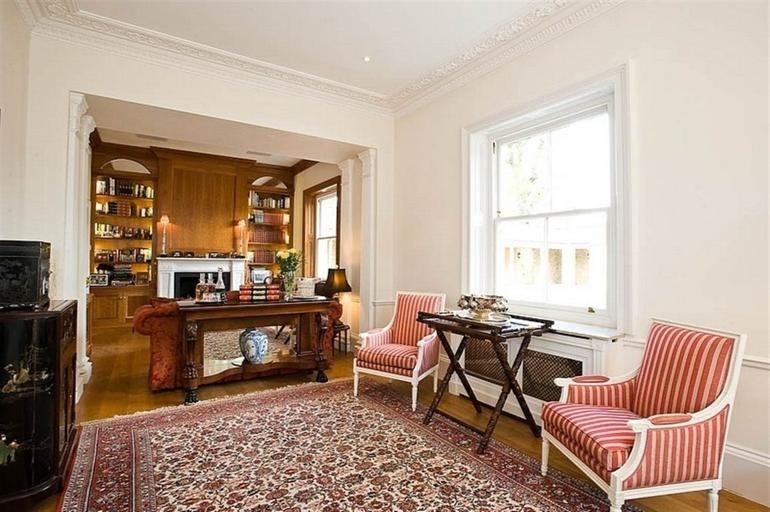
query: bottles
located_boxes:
[206,272,215,304]
[195,271,209,304]
[116,180,153,199]
[214,268,225,304]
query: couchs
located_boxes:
[130,296,344,395]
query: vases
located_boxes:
[279,270,297,302]
[237,326,271,365]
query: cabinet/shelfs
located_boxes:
[89,171,160,289]
[245,187,295,267]
[0,298,82,512]
[91,285,156,328]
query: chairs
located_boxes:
[351,289,447,411]
[538,318,748,511]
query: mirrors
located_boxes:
[203,324,297,377]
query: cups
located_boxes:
[203,292,213,301]
[211,292,220,302]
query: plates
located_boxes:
[457,313,511,327]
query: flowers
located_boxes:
[272,247,306,296]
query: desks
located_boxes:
[175,298,333,407]
[332,324,350,356]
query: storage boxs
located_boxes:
[0,237,54,314]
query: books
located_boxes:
[251,192,290,209]
[94,222,152,239]
[90,264,151,285]
[254,250,273,262]
[96,175,154,198]
[96,200,152,217]
[249,228,285,243]
[250,209,290,226]
[238,285,281,301]
[94,247,151,262]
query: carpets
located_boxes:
[52,372,660,512]
[204,327,291,359]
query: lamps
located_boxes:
[322,267,353,329]
